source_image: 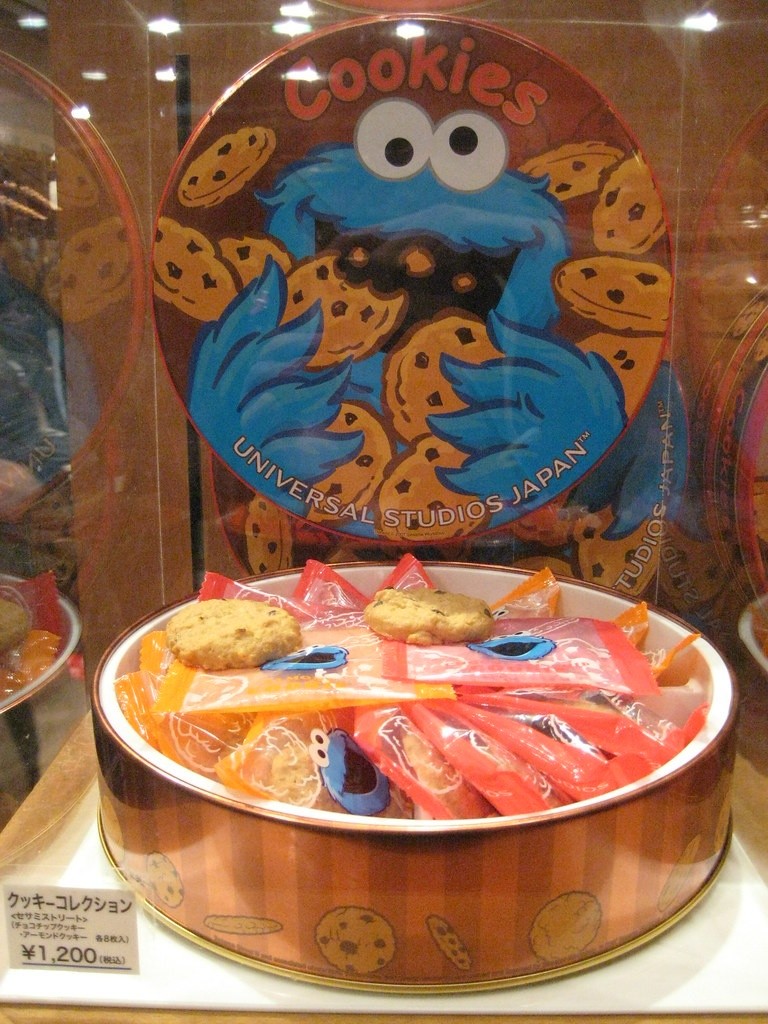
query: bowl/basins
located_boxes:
[0,574,82,716]
[738,594,768,673]
[95,559,741,993]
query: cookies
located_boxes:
[0,552,712,822]
